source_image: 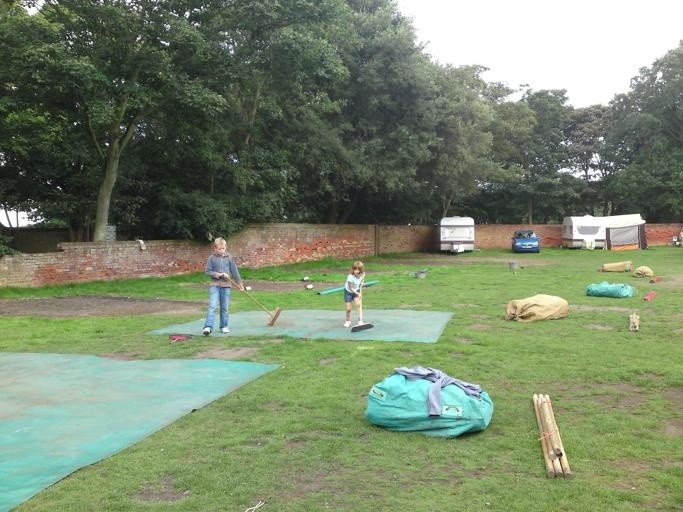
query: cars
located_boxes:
[509,227,540,254]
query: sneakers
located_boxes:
[221,327,229,333]
[343,321,364,327]
[203,327,211,336]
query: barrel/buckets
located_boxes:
[415,271,426,280]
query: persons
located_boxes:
[201,236,245,336]
[342,259,366,329]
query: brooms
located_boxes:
[169,335,192,344]
[350,286,374,332]
[224,276,282,326]
